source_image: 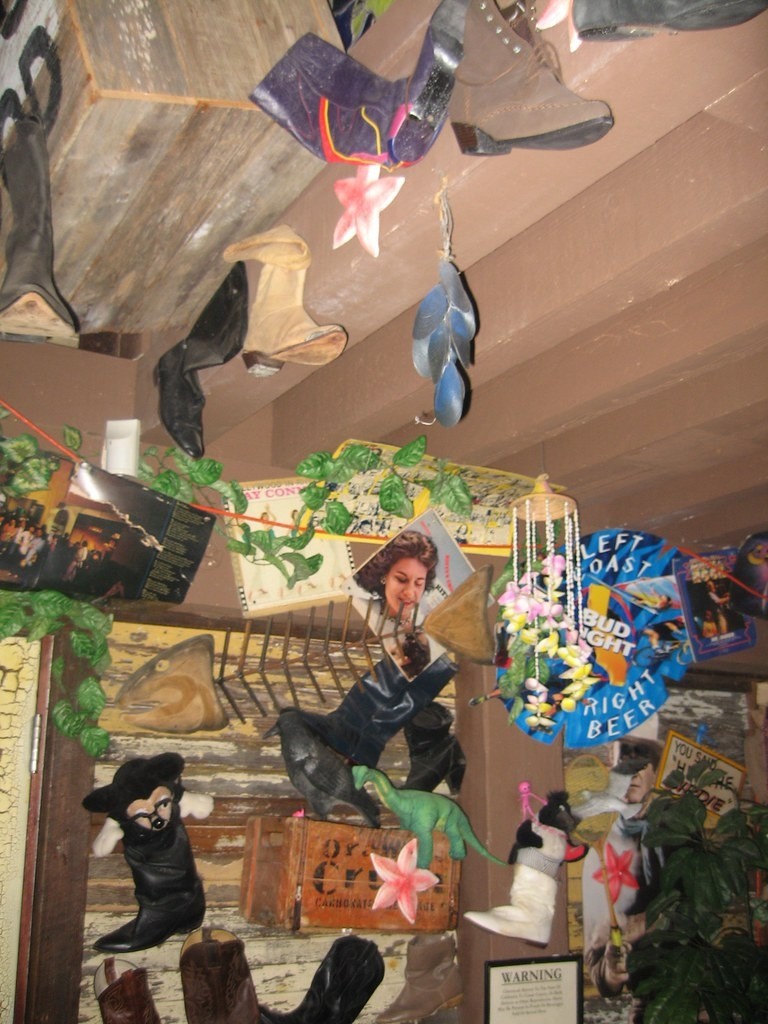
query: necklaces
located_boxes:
[381,609,411,625]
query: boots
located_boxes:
[374,929,464,1024]
[179,927,261,1024]
[249,0,473,170]
[280,642,459,769]
[395,701,466,794]
[0,114,75,337]
[152,261,249,459]
[259,935,385,1024]
[82,753,207,953]
[225,224,349,378]
[445,0,615,156]
[93,957,162,1024]
[463,789,589,949]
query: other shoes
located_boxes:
[571,0,768,41]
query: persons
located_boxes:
[701,581,743,639]
[622,743,660,805]
[631,584,671,608]
[355,531,440,654]
[0,506,106,597]
[641,615,686,655]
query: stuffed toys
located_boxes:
[81,752,215,858]
[507,790,589,865]
